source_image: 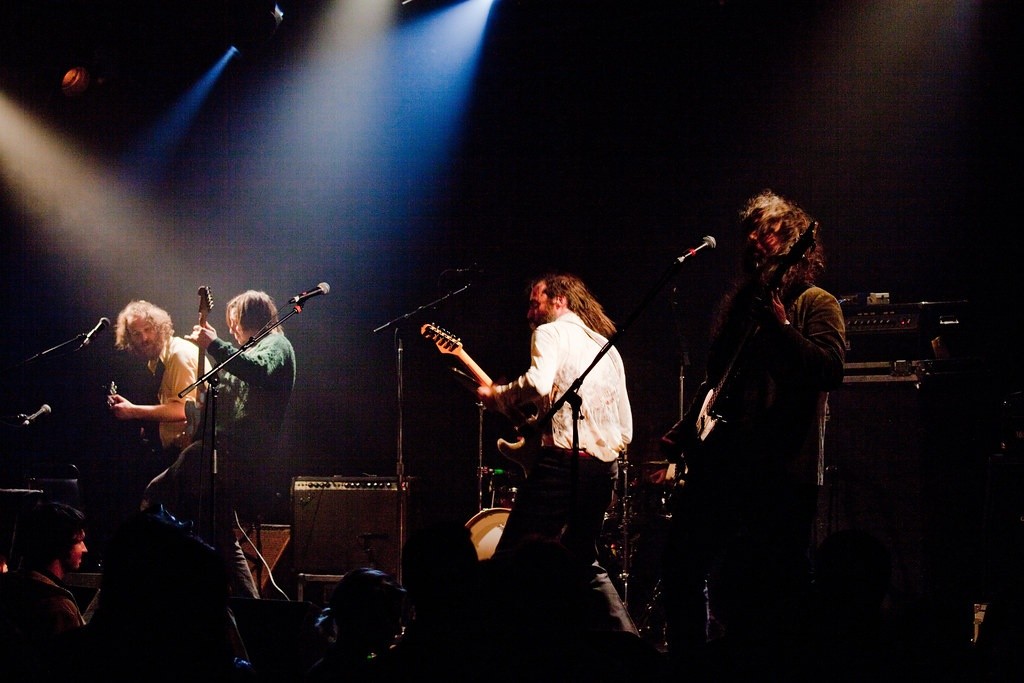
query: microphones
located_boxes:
[22,405,52,426]
[673,236,717,265]
[453,265,485,273]
[287,282,330,304]
[79,317,111,350]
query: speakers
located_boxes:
[229,475,420,682]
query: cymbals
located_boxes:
[449,366,489,403]
[641,460,672,466]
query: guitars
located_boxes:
[99,380,182,477]
[194,283,215,436]
[662,219,822,475]
[419,318,553,476]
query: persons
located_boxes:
[662,192,845,656]
[142,291,296,599]
[109,301,212,482]
[0,466,1024,683]
[475,271,634,578]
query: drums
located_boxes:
[462,504,513,562]
